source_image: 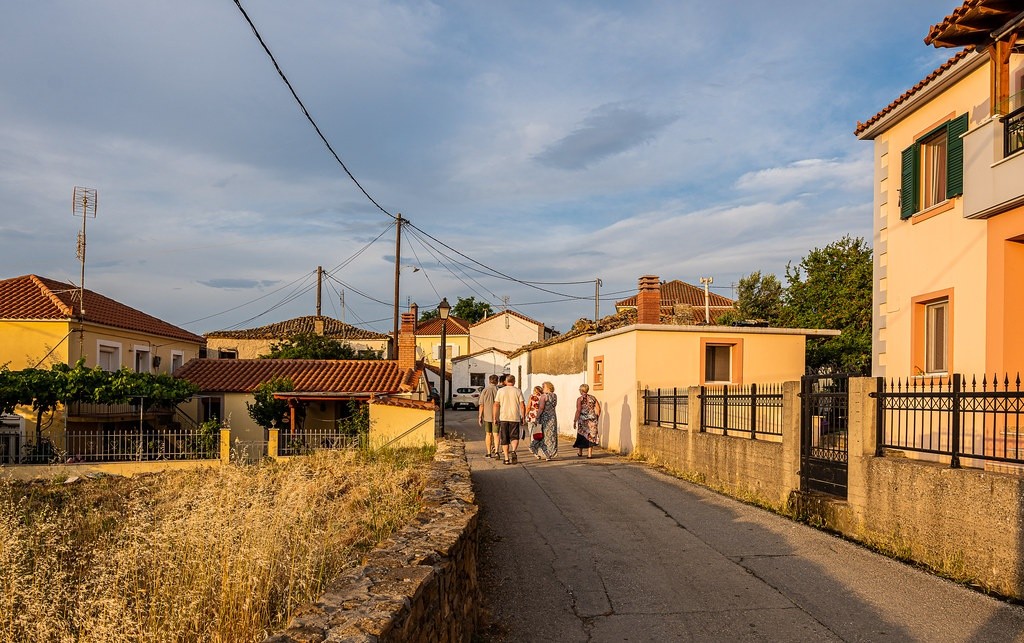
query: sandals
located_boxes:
[484,454,492,458]
[511,451,517,464]
[494,452,500,460]
[502,459,510,465]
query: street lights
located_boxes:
[394,266,420,358]
[437,297,451,437]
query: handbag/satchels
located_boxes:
[532,423,543,441]
[520,425,526,441]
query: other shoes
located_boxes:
[577,451,582,456]
[537,455,541,459]
[587,456,593,458]
[546,458,550,461]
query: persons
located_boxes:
[493,375,526,462]
[572,384,601,459]
[498,376,507,388]
[530,381,558,461]
[525,386,543,454]
[478,375,501,460]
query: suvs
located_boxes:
[452,386,485,411]
